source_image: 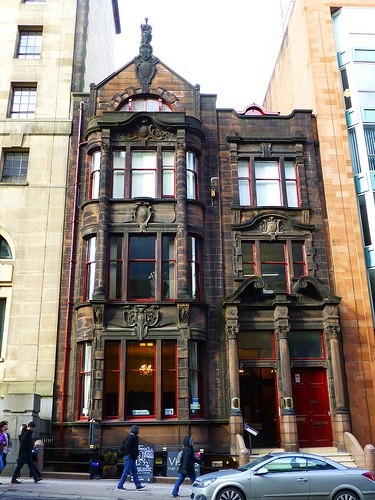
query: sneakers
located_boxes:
[116,486,128,492]
[136,485,147,491]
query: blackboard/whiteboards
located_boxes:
[129,443,155,483]
[166,451,200,478]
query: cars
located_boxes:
[190,450,375,500]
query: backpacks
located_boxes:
[174,450,184,467]
[117,437,127,458]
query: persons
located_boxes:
[0,420,12,485]
[171,435,206,497]
[10,422,43,484]
[118,425,146,490]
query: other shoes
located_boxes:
[34,478,42,482]
[11,480,21,484]
[171,494,180,498]
